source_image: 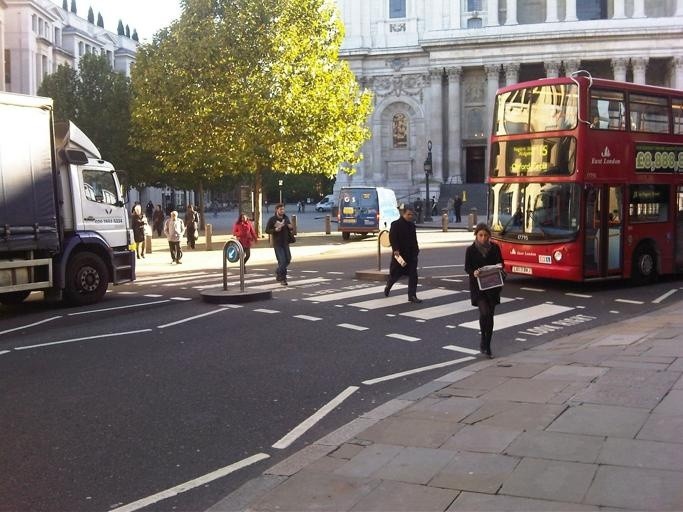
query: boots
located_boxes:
[480,314,494,355]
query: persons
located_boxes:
[232,213,257,275]
[301,200,305,213]
[447,197,454,223]
[182,205,199,250]
[413,197,422,210]
[264,204,294,286]
[453,194,462,223]
[130,198,164,259]
[463,222,505,358]
[384,205,422,304]
[431,196,438,216]
[297,200,301,212]
[163,211,185,264]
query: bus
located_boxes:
[480,70,683,286]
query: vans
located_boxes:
[315,194,334,213]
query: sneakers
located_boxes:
[408,296,422,303]
[276,277,287,285]
[385,286,389,297]
[137,253,145,259]
[171,259,182,264]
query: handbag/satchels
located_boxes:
[288,231,296,244]
[194,229,198,240]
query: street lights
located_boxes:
[277,179,283,204]
[423,157,433,221]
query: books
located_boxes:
[274,219,284,230]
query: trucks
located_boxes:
[336,186,400,241]
[0,92,138,309]
